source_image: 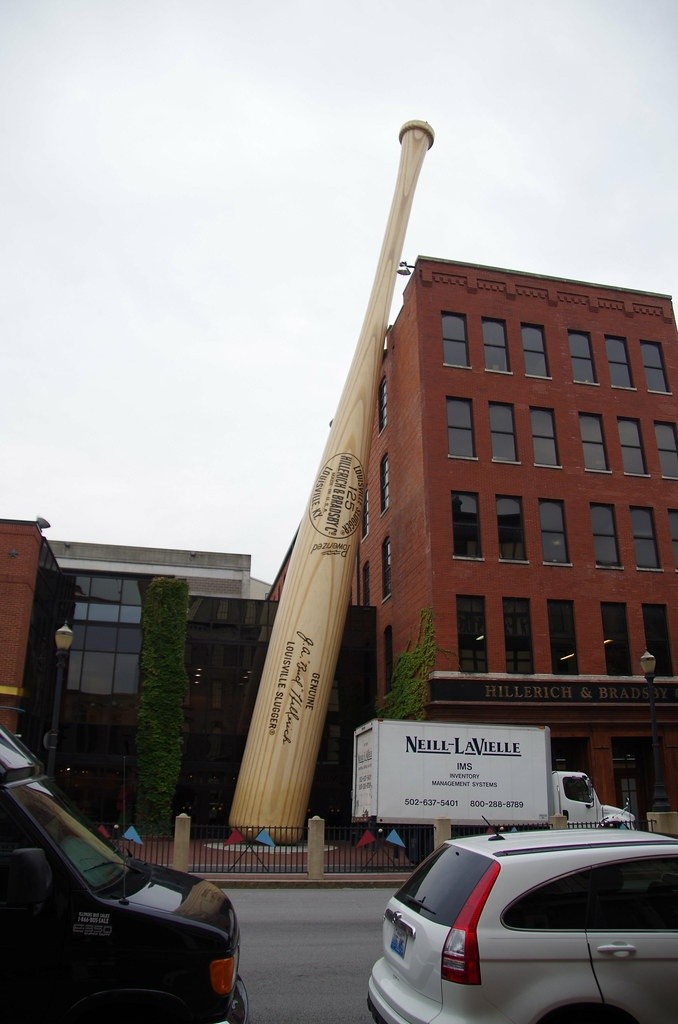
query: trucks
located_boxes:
[350,717,636,869]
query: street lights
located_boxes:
[641,645,678,838]
[47,618,75,782]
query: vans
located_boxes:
[0,721,248,1024]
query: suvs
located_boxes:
[366,827,678,1024]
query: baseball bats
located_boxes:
[224,120,438,842]
